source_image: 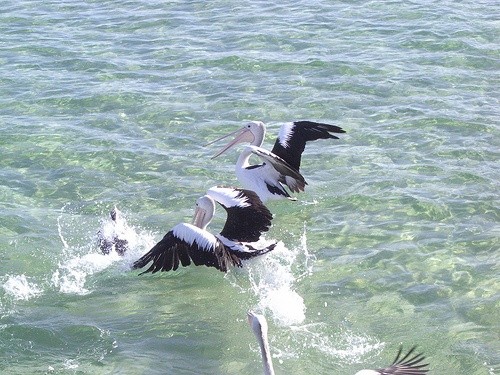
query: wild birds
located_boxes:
[247,312,431,375]
[203,118,347,205]
[130,186,279,275]
